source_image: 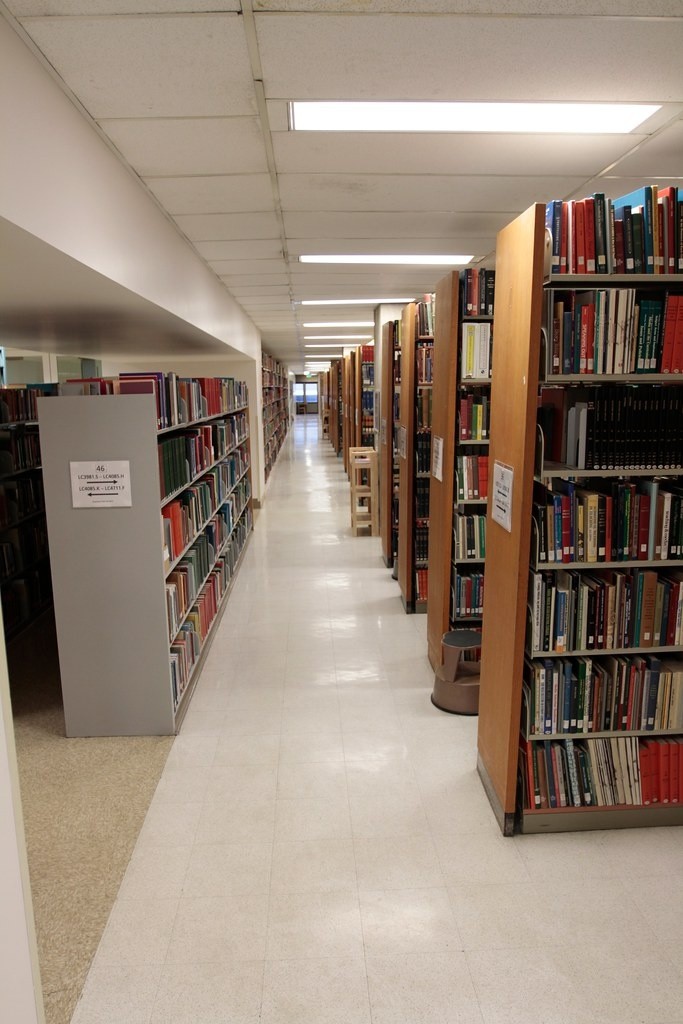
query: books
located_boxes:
[516,182,683,814]
[0,370,256,647]
[317,319,398,563]
[261,348,294,486]
[411,293,440,608]
[449,264,504,666]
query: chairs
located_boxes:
[297,402,305,415]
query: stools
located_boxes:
[430,630,482,717]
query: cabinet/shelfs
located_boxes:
[477,205,683,837]
[261,350,294,485]
[397,292,438,614]
[348,444,380,536]
[356,345,374,500]
[348,351,356,485]
[426,268,494,663]
[0,382,59,647]
[317,361,342,457]
[35,372,256,741]
[381,320,401,568]
